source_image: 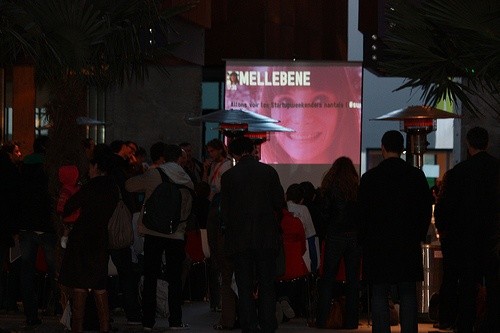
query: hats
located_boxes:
[232,135,254,148]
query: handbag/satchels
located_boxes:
[107,192,135,249]
[302,236,320,272]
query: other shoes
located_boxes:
[7,310,18,315]
[127,322,143,325]
[209,306,223,312]
[212,323,233,330]
[167,320,190,329]
[24,318,44,327]
[275,301,283,323]
[281,300,294,318]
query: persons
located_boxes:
[225,73,246,100]
[0,126,500,333]
[262,67,361,164]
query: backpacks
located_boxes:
[141,167,197,234]
